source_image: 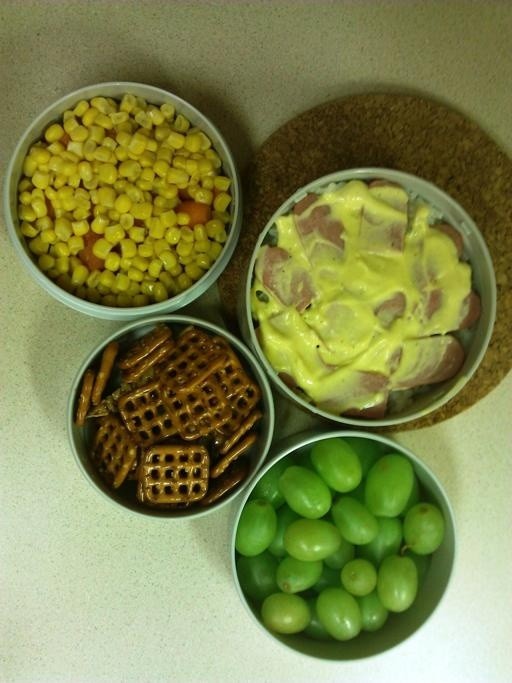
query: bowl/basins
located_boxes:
[3,80,241,321]
[238,169,496,425]
[228,430,458,663]
[68,315,276,521]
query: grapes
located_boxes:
[233,438,446,642]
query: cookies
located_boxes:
[71,324,263,512]
